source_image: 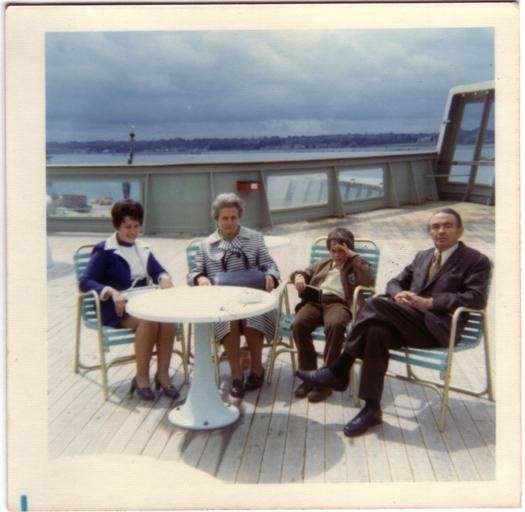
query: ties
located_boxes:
[427,250,442,286]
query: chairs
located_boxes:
[186,236,299,390]
[265,237,380,398]
[72,242,190,403]
[356,258,495,432]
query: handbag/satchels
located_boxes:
[119,275,162,302]
[212,246,267,291]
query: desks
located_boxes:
[124,285,280,432]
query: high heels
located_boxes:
[129,376,155,401]
[154,371,179,398]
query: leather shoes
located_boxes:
[294,366,350,392]
[230,376,245,399]
[294,378,316,398]
[307,386,333,403]
[243,366,265,390]
[343,407,383,438]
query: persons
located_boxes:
[293,208,491,438]
[78,199,180,401]
[289,227,375,402]
[187,191,280,398]
